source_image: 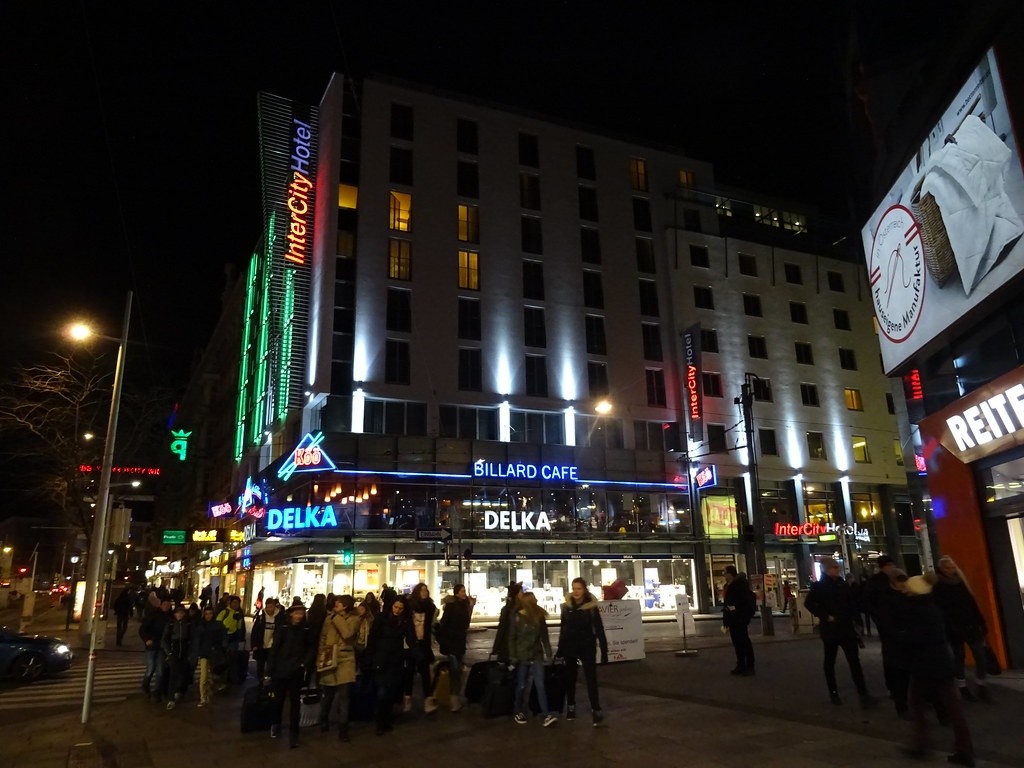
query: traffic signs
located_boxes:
[415,527,452,541]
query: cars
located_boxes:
[0,625,74,684]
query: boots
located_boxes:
[424,697,438,714]
[338,721,350,741]
[320,715,329,731]
[403,693,412,712]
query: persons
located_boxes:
[250,583,478,747]
[780,581,791,613]
[845,553,990,768]
[802,558,878,707]
[113,586,133,648]
[555,576,608,727]
[253,586,265,621]
[199,584,220,608]
[137,593,249,709]
[129,580,187,617]
[723,566,758,676]
[491,581,559,726]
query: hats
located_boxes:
[287,601,307,612]
[819,557,836,572]
[904,571,937,595]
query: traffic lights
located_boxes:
[343,548,350,563]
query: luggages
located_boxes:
[241,678,280,733]
[300,670,324,728]
[529,655,567,713]
[465,650,500,703]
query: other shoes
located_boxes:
[141,677,209,709]
[947,753,975,768]
[731,665,743,674]
[289,733,300,747]
[831,693,843,705]
[743,666,755,676]
[859,696,880,705]
[542,715,559,727]
[960,687,978,703]
[592,712,601,727]
[566,705,576,720]
[513,713,527,724]
[270,724,280,738]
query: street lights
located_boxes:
[67,290,140,634]
[66,552,80,630]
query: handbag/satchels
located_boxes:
[315,644,338,673]
